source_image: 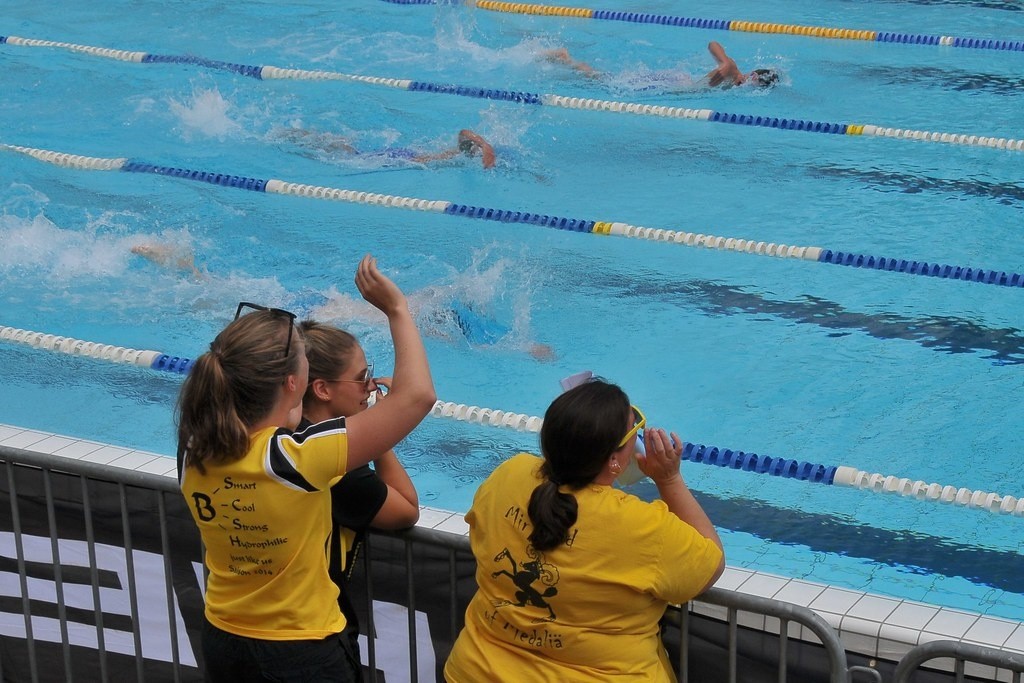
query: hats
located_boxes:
[756,66,781,88]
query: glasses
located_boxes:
[614,403,646,449]
[306,360,375,390]
[232,301,297,385]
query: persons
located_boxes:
[177,254,437,683]
[439,381,725,683]
[296,320,420,683]
[707,41,779,87]
[458,130,495,167]
